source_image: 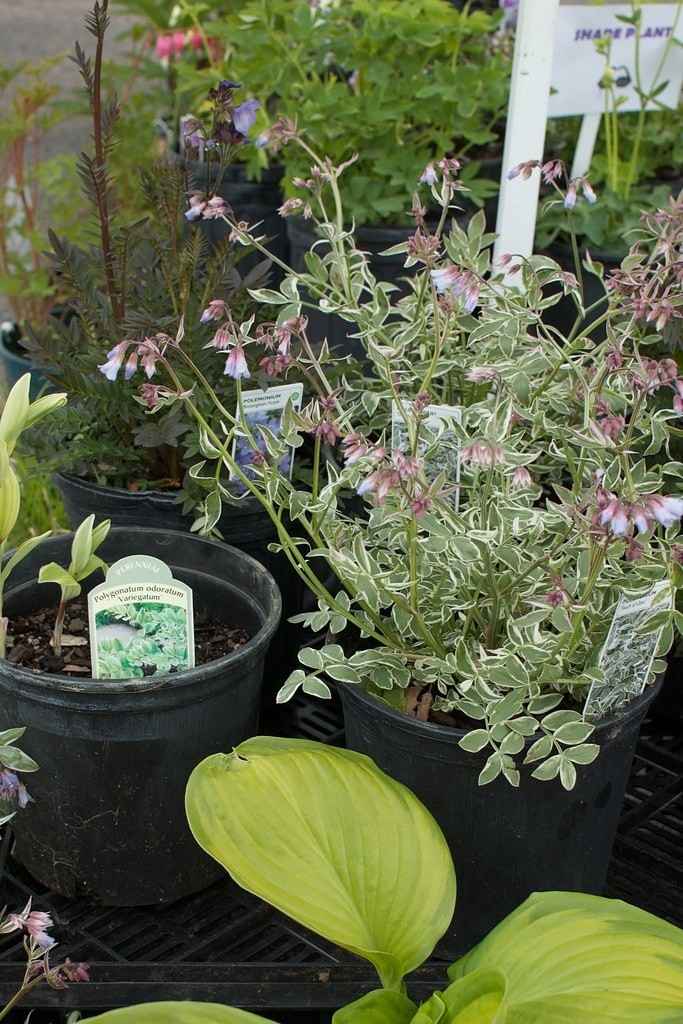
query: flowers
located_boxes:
[100,78,682,793]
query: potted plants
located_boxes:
[0,0,683,906]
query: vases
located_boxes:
[324,610,663,959]
